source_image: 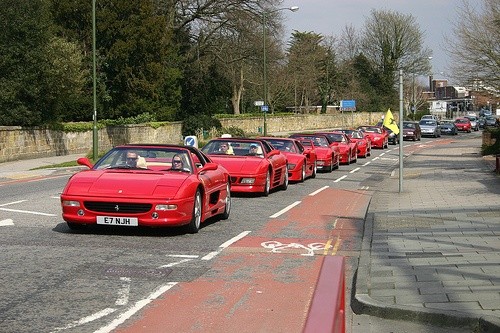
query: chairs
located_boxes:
[136,157,147,170]
[225,144,235,155]
[250,143,264,155]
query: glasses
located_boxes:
[172,159,182,163]
[126,157,137,161]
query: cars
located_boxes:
[397,121,420,141]
[334,129,372,157]
[377,122,399,145]
[453,118,471,133]
[464,108,497,131]
[324,132,358,165]
[259,137,317,181]
[422,115,438,122]
[291,133,340,172]
[199,137,289,196]
[419,119,441,138]
[438,122,457,135]
[357,126,389,149]
[59,143,232,233]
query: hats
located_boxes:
[250,143,258,147]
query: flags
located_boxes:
[382,108,400,135]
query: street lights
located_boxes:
[261,6,301,137]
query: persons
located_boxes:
[116,150,139,168]
[171,153,190,171]
[218,142,235,155]
[246,143,263,157]
